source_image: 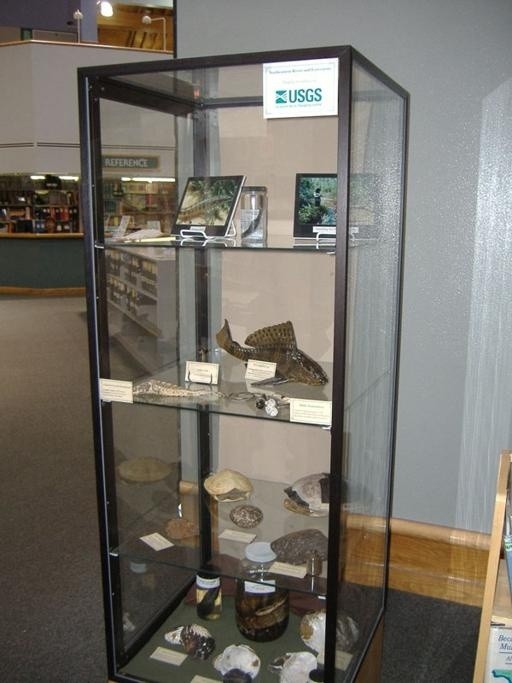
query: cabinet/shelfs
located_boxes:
[0,175,178,234]
[75,45,412,682]
[105,237,179,376]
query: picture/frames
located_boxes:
[292,172,386,249]
[169,174,248,242]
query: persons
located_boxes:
[39,216,60,233]
[313,188,324,207]
